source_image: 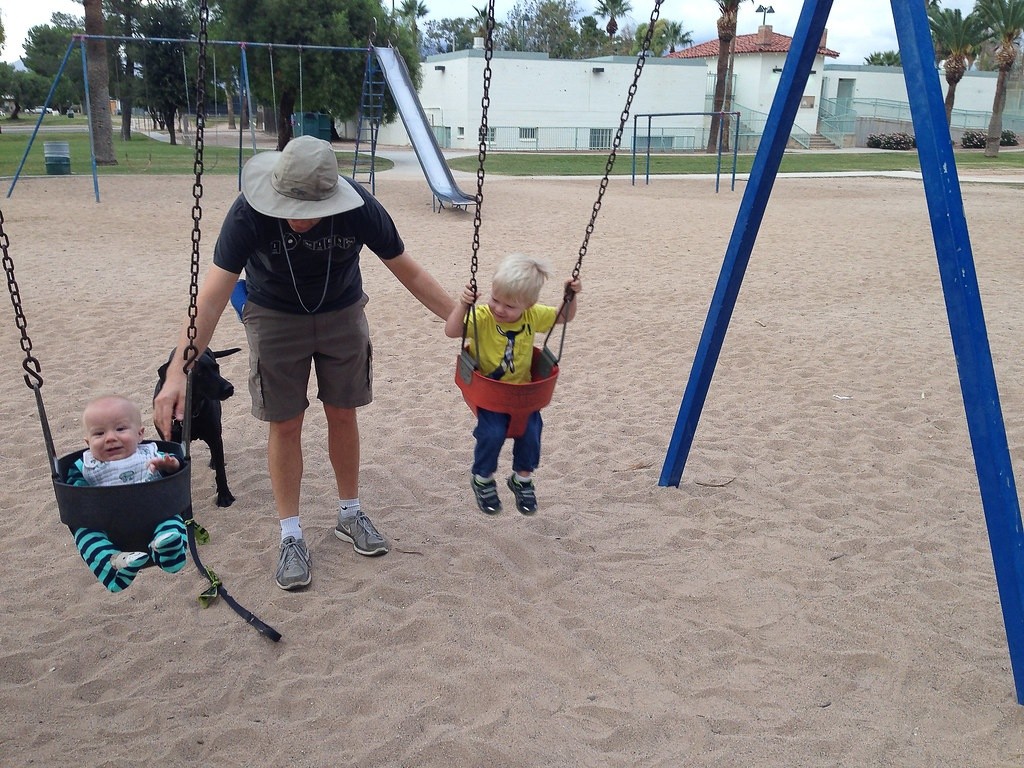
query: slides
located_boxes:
[372,46,481,206]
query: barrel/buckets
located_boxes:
[43,141,70,175]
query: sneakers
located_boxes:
[507,474,538,516]
[334,507,389,556]
[275,529,313,591]
[469,473,503,515]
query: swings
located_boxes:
[0,0,211,573]
[453,0,666,440]
[112,38,152,174]
[180,41,221,172]
[268,44,304,152]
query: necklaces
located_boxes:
[278,216,335,314]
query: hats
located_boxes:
[240,134,365,220]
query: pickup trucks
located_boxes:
[25,106,53,115]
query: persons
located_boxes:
[153,134,470,590]
[445,256,581,514]
[65,396,188,593]
